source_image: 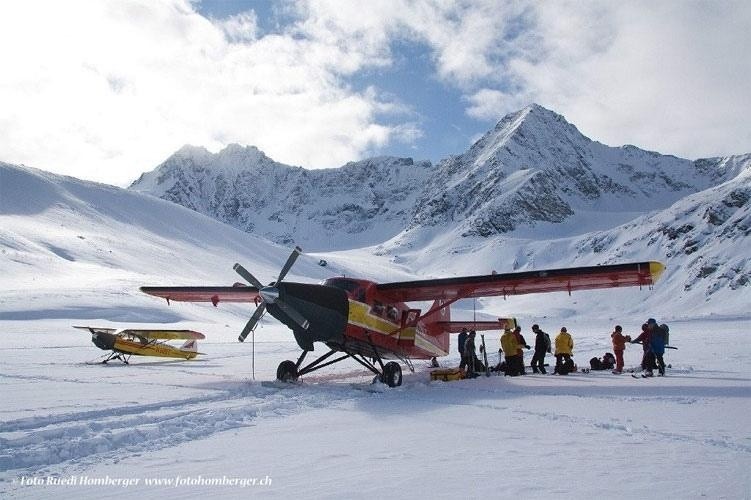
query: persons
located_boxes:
[464,332,478,378]
[613,319,672,377]
[457,328,470,370]
[501,325,574,376]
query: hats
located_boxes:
[615,325,622,330]
[532,324,539,329]
[462,327,476,335]
[561,327,567,332]
[642,324,648,330]
[504,325,521,332]
[646,319,656,323]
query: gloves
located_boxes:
[525,345,530,350]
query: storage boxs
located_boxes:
[430,368,462,382]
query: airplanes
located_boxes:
[139,246,664,387]
[72,324,208,368]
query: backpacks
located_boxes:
[557,359,575,375]
